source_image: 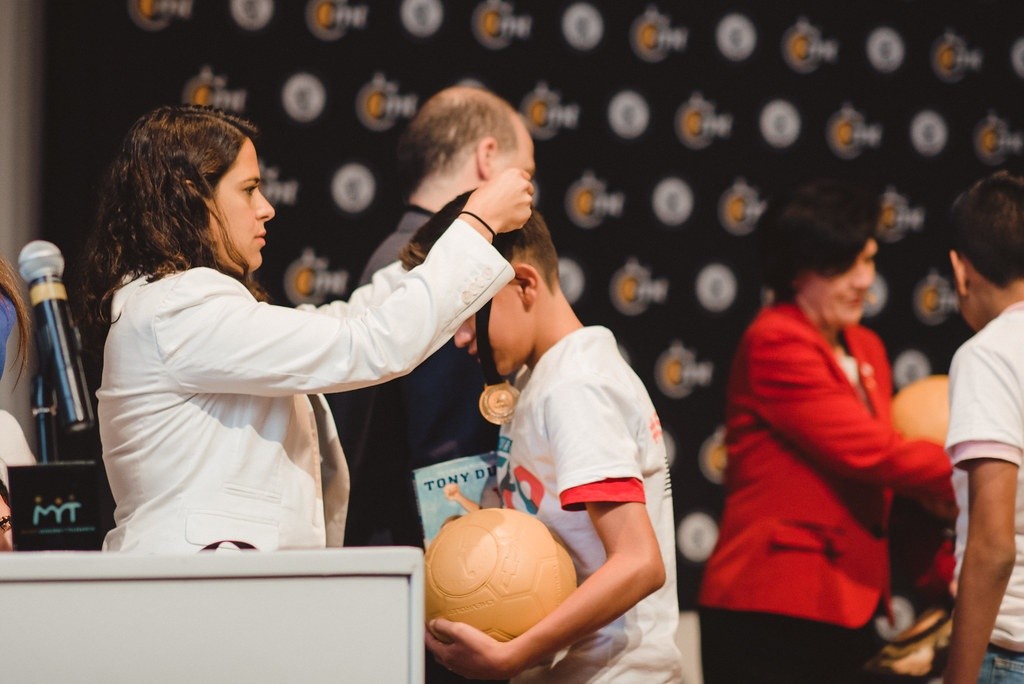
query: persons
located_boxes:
[94,103,535,552]
[888,171,1024,684]
[698,186,958,684]
[0,256,32,551]
[356,83,679,683]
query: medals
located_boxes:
[478,383,522,426]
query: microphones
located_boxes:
[19,241,94,431]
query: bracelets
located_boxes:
[457,210,496,235]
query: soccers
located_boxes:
[418,504,585,658]
